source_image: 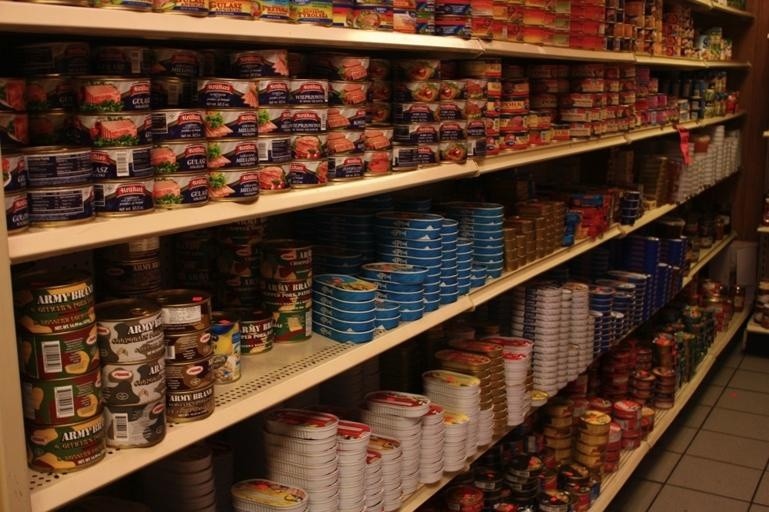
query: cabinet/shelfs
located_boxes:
[741,120,769,353]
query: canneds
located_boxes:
[435,1,605,49]
[214,219,313,385]
[394,57,530,172]
[14,237,213,474]
[262,49,394,195]
[91,0,435,34]
[607,0,734,59]
[0,40,261,235]
[676,70,740,123]
[531,62,667,143]
